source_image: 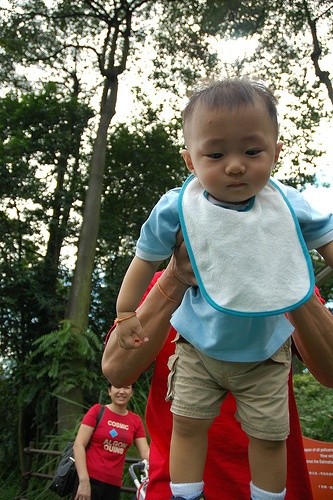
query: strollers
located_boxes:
[128,461,149,500]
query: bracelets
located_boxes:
[104,313,135,345]
[155,279,181,303]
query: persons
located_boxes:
[101,232,333,500]
[73,382,150,500]
[115,79,333,500]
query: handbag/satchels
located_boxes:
[49,445,79,497]
